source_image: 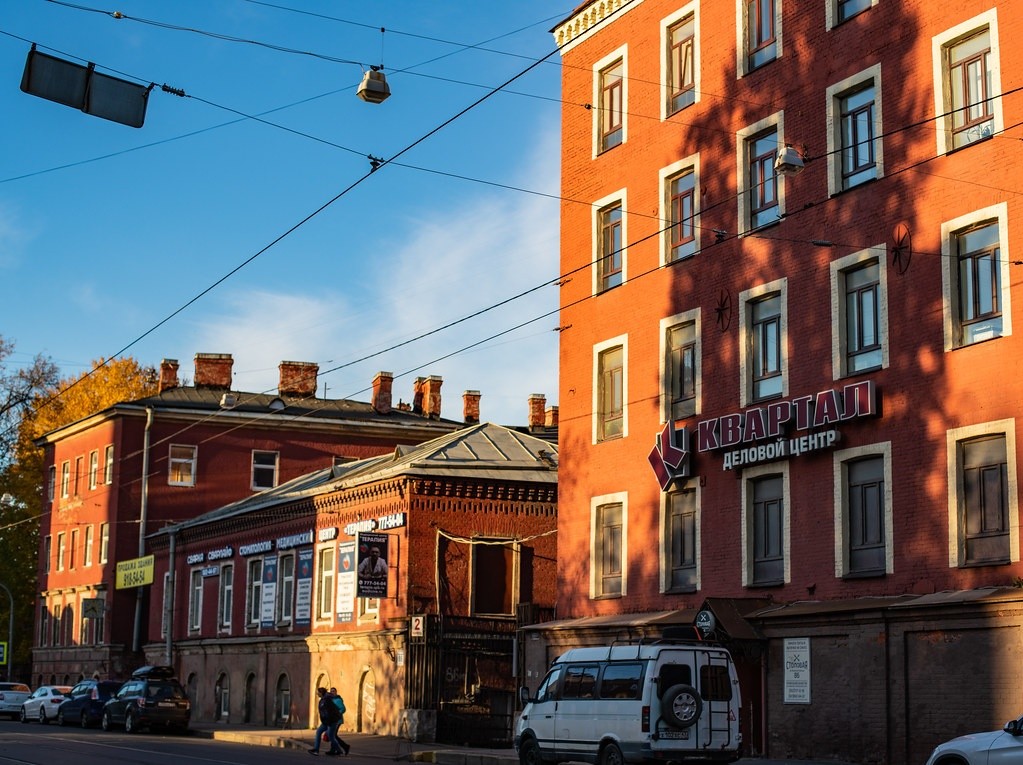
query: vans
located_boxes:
[515,627,745,765]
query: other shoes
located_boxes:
[335,751,340,755]
[308,749,319,756]
[326,750,335,755]
[345,745,349,755]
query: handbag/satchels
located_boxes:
[323,733,329,742]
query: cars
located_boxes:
[927,715,1023,765]
[0,682,32,721]
[20,685,75,725]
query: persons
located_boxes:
[358,545,388,579]
[307,687,345,757]
[325,687,350,755]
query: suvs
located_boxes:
[102,666,191,735]
[57,679,125,729]
